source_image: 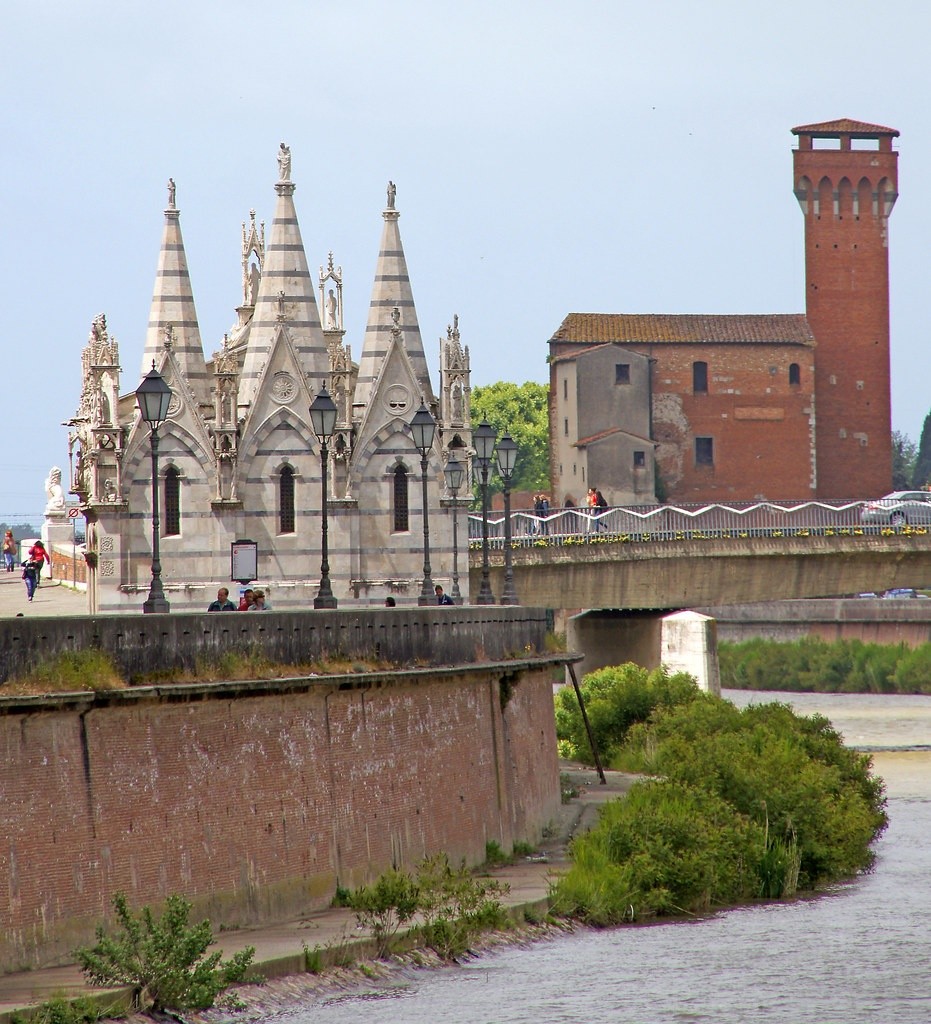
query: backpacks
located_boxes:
[2,542,10,554]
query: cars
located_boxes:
[861,491,931,528]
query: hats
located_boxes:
[29,556,36,560]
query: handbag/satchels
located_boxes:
[21,568,27,579]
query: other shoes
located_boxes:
[7,567,13,572]
[593,531,599,534]
[604,524,607,529]
[28,596,32,603]
[36,581,39,584]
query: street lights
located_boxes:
[307,379,341,610]
[471,412,497,607]
[493,428,519,607]
[408,396,443,606]
[134,359,172,614]
[443,451,465,605]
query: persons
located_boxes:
[2,531,15,572]
[208,588,237,612]
[167,178,176,204]
[435,585,454,605]
[249,263,260,302]
[277,143,291,180]
[326,289,336,329]
[387,181,396,207]
[28,541,50,584]
[587,488,608,533]
[21,556,39,601]
[385,597,395,607]
[525,495,548,536]
[237,589,272,610]
